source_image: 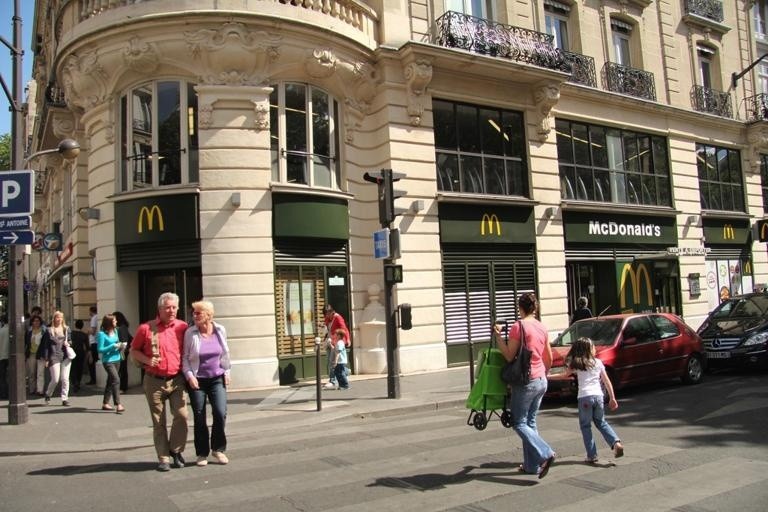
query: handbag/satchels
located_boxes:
[63,340,77,360]
[119,342,125,361]
[501,347,534,387]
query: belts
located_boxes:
[145,371,182,381]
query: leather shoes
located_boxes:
[102,406,116,410]
[117,407,126,413]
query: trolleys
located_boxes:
[464,320,516,432]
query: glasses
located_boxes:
[192,311,204,316]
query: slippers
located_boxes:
[538,452,556,479]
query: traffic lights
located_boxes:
[400,303,412,331]
[383,264,402,283]
[387,171,408,224]
[363,168,391,225]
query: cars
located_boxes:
[696,292,767,373]
[539,311,705,403]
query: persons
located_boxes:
[0,306,133,412]
[569,296,593,326]
[323,304,350,390]
[129,292,190,471]
[493,292,556,479]
[327,329,350,390]
[181,300,231,466]
[547,337,624,463]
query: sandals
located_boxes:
[613,441,625,458]
[583,456,600,463]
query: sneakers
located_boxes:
[196,455,208,466]
[63,400,72,406]
[211,450,229,465]
[44,393,51,402]
[169,450,186,468]
[157,460,170,472]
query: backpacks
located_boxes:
[128,319,160,370]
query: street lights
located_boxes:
[4,139,82,424]
